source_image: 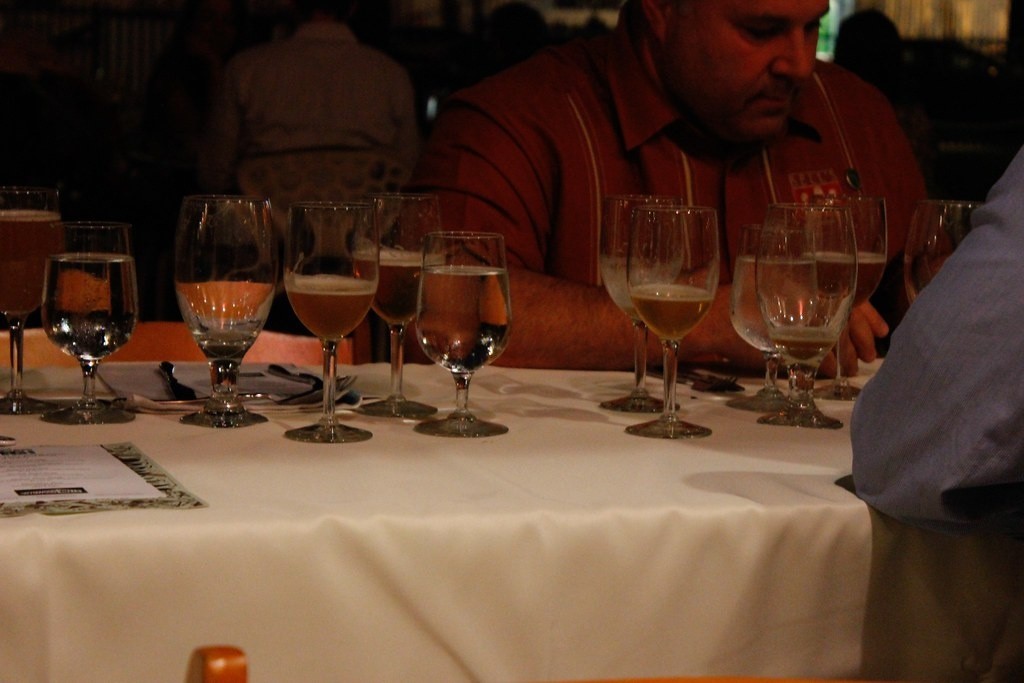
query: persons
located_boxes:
[0,0,611,324]
[851,132,1024,543]
[384,1,951,385]
[822,6,1024,338]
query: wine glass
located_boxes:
[0,182,137,424]
[285,199,376,445]
[363,190,439,420]
[600,190,722,441]
[727,192,887,432]
[904,197,981,309]
[414,229,512,437]
[175,193,278,427]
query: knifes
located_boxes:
[163,354,198,401]
[652,365,745,395]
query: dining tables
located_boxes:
[1,358,891,683]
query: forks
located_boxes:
[267,362,358,396]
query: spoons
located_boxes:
[146,378,347,407]
